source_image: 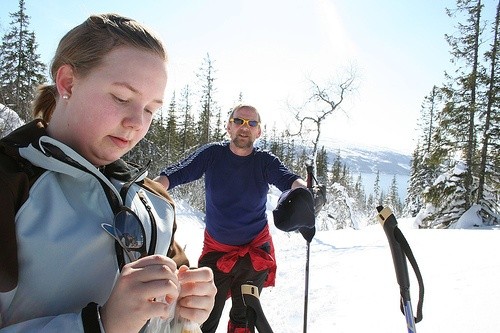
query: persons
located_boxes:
[0,11,218,333]
[153,104,308,333]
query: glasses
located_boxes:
[100,204,149,263]
[229,117,260,127]
